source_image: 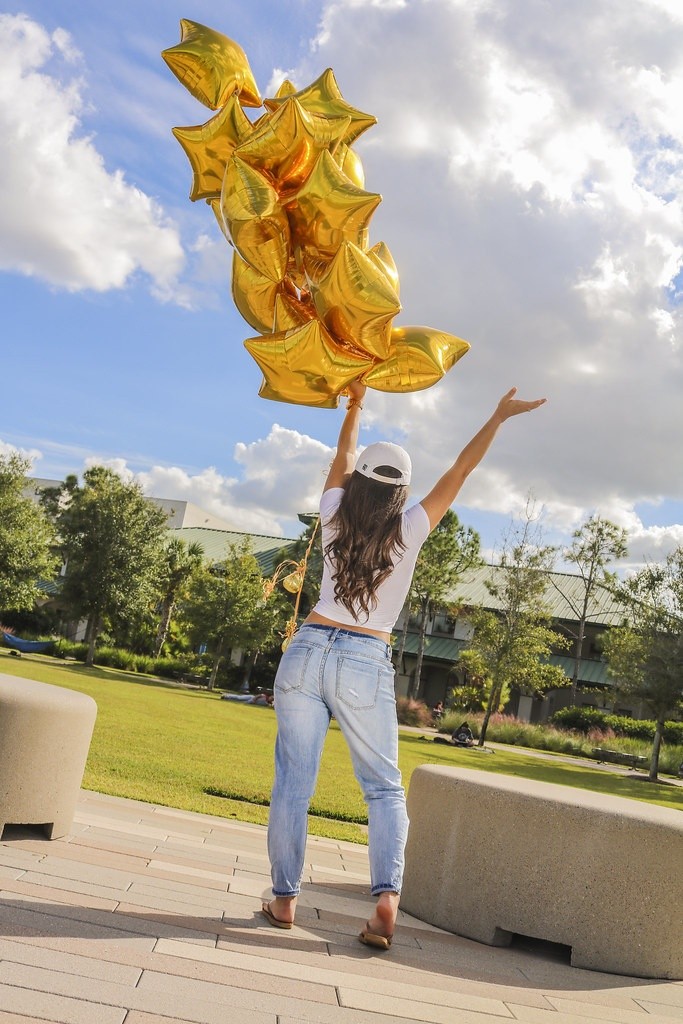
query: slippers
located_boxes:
[261,901,293,929]
[357,921,393,950]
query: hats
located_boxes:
[355,442,412,486]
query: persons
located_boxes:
[431,700,444,721]
[451,721,475,747]
[260,373,547,950]
[220,693,274,707]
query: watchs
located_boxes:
[345,398,364,410]
